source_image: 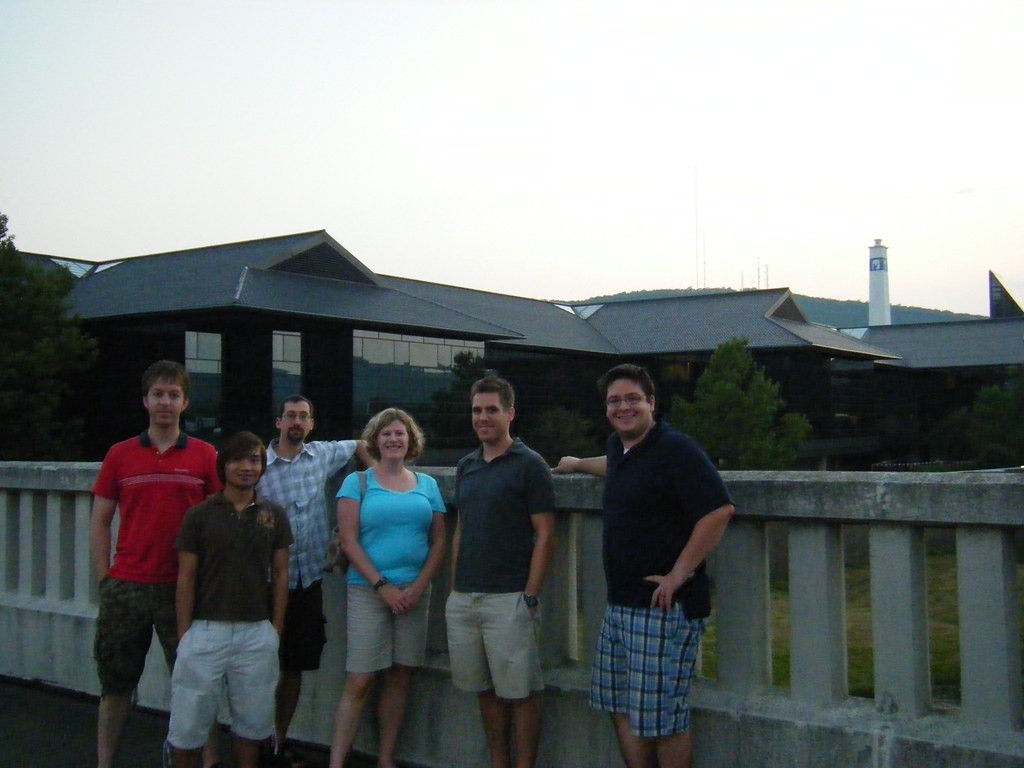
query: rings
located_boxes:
[659,594,665,597]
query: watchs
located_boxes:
[523,593,539,607]
[372,576,388,591]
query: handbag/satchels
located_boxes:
[322,469,366,574]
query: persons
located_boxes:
[166,430,296,768]
[327,405,447,768]
[254,394,377,768]
[548,363,736,768]
[89,360,224,768]
[446,373,557,768]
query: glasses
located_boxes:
[602,391,650,407]
[281,411,311,422]
